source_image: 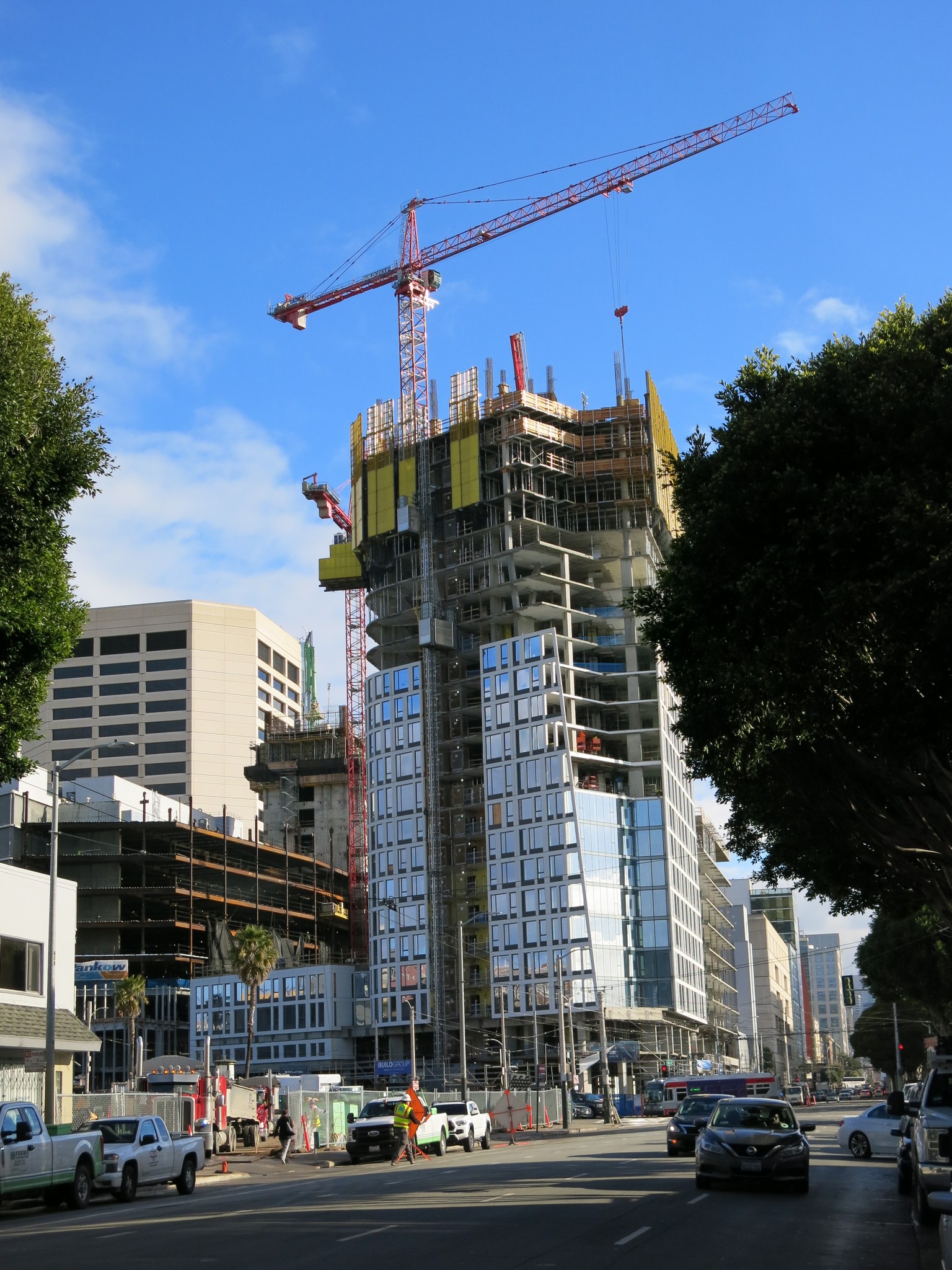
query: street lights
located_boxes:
[554,993,582,1091]
[734,1016,760,1073]
[86,1007,109,1106]
[485,1038,511,1066]
[556,947,592,1129]
[405,1000,417,1091]
[41,738,138,1126]
[458,911,504,1103]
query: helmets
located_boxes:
[401,1094,411,1101]
[310,1099,315,1104]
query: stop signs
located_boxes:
[412,1080,419,1090]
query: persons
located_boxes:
[768,1110,784,1129]
[391,1094,422,1165]
[305,1098,326,1149]
[273,1108,295,1164]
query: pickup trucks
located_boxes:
[428,1098,492,1152]
[345,1092,450,1161]
[0,1102,105,1211]
[67,1116,206,1203]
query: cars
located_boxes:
[826,1092,839,1103]
[666,1093,737,1156]
[693,1097,815,1197]
[838,1081,885,1101]
[837,1101,920,1160]
[890,1046,952,1270]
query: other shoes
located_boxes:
[391,1162,401,1166]
[281,1159,286,1164]
[411,1160,416,1164]
[280,1156,288,1162]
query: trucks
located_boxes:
[236,1069,343,1142]
[782,1081,832,1106]
[107,1035,260,1152]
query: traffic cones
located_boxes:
[805,1095,810,1107]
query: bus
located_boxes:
[638,1072,781,1116]
[841,1077,866,1089]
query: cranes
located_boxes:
[267,91,802,447]
[302,471,372,963]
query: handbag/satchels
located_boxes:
[287,1118,295,1136]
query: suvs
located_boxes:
[570,1092,616,1118]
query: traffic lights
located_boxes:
[797,1078,799,1082]
[899,1045,903,1049]
[841,975,856,1006]
[661,1065,669,1077]
